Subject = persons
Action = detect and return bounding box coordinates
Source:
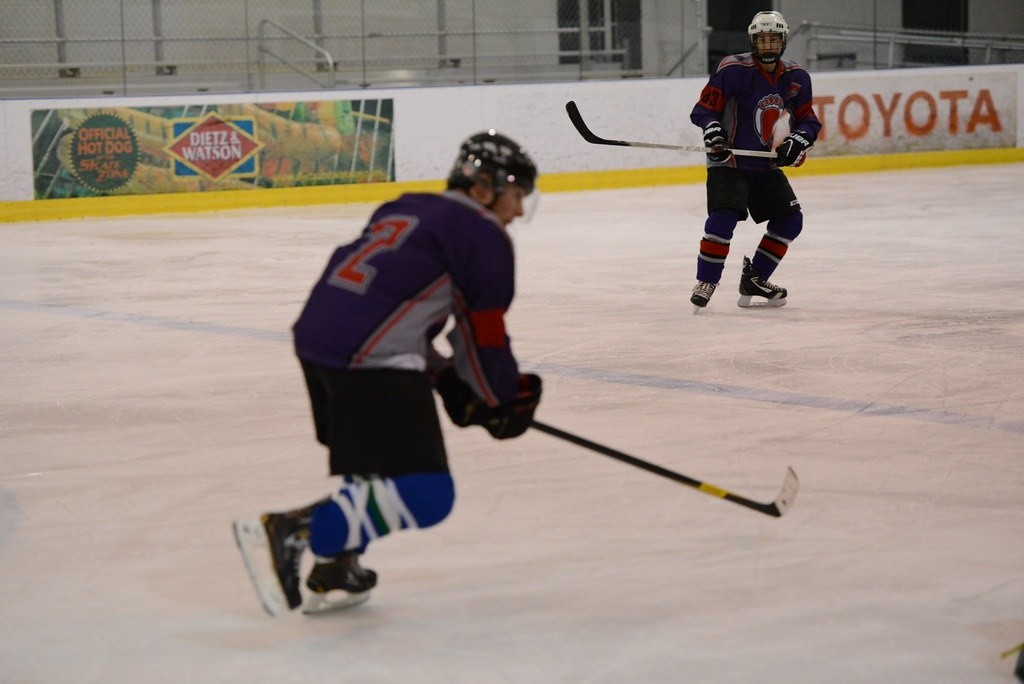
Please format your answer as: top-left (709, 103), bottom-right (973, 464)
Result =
top-left (233, 132), bottom-right (543, 619)
top-left (689, 11), bottom-right (823, 317)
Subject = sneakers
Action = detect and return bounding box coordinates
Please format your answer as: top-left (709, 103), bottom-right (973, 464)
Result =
top-left (689, 280), bottom-right (716, 308)
top-left (300, 553), bottom-right (379, 614)
top-left (232, 512), bottom-right (310, 615)
top-left (737, 255), bottom-right (788, 308)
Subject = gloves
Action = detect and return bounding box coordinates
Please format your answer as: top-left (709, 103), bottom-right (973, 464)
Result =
top-left (702, 120), bottom-right (733, 164)
top-left (431, 356), bottom-right (484, 428)
top-left (484, 372), bottom-right (543, 439)
top-left (775, 129), bottom-right (814, 168)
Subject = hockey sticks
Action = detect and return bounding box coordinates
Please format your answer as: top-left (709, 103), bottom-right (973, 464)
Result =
top-left (527, 417), bottom-right (799, 519)
top-left (565, 98), bottom-right (778, 159)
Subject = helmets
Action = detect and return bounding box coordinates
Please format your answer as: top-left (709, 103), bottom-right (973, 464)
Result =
top-left (447, 131), bottom-right (538, 196)
top-left (748, 10), bottom-right (791, 65)
